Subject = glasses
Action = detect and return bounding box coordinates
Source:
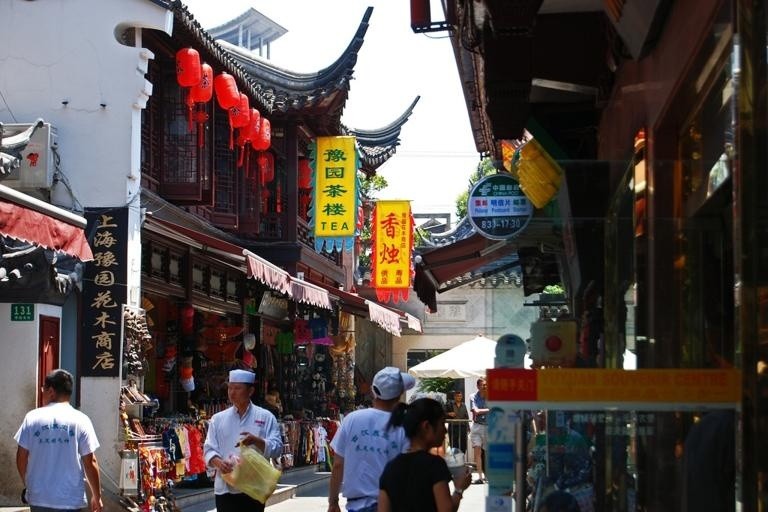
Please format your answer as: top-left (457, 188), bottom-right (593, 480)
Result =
top-left (40, 385), bottom-right (45, 393)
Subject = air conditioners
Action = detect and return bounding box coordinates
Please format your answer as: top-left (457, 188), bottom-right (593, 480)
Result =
top-left (0, 122), bottom-right (59, 191)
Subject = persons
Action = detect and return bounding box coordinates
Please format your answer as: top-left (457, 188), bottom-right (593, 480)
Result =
top-left (442, 390), bottom-right (471, 457)
top-left (467, 376), bottom-right (490, 484)
top-left (372, 395), bottom-right (474, 512)
top-left (532, 489), bottom-right (581, 511)
top-left (325, 366), bottom-right (418, 512)
top-left (8, 368), bottom-right (104, 511)
top-left (200, 368), bottom-right (285, 512)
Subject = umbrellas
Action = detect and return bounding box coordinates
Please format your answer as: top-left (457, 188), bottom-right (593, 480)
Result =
top-left (406, 332), bottom-right (535, 384)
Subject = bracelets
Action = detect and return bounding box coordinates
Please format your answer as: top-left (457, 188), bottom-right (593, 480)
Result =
top-left (328, 497), bottom-right (339, 505)
top-left (454, 490), bottom-right (463, 499)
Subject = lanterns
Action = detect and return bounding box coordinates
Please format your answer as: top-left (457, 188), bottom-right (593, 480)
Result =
top-left (174, 46), bottom-right (201, 132)
top-left (214, 70), bottom-right (240, 152)
top-left (255, 151), bottom-right (275, 217)
top-left (353, 205), bottom-right (365, 255)
top-left (297, 157), bottom-right (313, 221)
top-left (228, 91), bottom-right (250, 168)
top-left (188, 61), bottom-right (214, 149)
top-left (249, 117), bottom-right (271, 189)
top-left (237, 107), bottom-right (262, 178)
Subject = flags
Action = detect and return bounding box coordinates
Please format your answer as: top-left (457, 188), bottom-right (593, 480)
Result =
top-left (307, 135), bottom-right (363, 256)
top-left (370, 199), bottom-right (416, 304)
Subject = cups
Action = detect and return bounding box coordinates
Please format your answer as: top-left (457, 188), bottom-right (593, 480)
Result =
top-left (442, 446), bottom-right (467, 479)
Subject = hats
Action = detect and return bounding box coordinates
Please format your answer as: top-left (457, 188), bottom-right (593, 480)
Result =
top-left (242, 333), bottom-right (255, 352)
top-left (160, 307), bottom-right (195, 392)
top-left (228, 369), bottom-right (255, 384)
top-left (371, 366), bottom-right (416, 400)
